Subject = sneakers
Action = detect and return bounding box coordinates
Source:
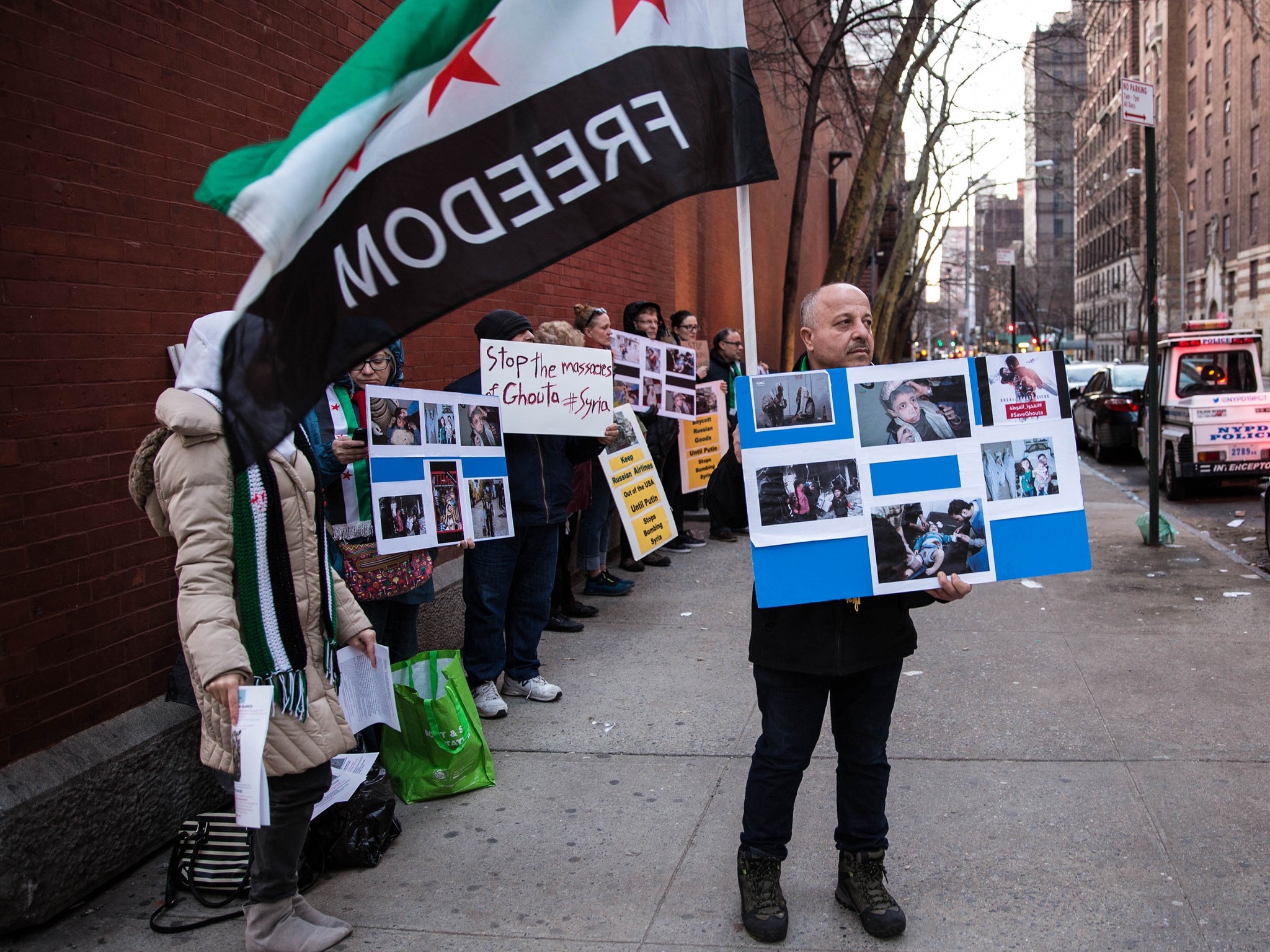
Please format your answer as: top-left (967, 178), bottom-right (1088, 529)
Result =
top-left (470, 681), bottom-right (508, 720)
top-left (835, 848), bottom-right (907, 939)
top-left (737, 842), bottom-right (789, 942)
top-left (500, 670), bottom-right (563, 703)
top-left (583, 572), bottom-right (632, 596)
top-left (678, 530), bottom-right (707, 547)
top-left (658, 538), bottom-right (692, 553)
top-left (601, 569), bottom-right (636, 588)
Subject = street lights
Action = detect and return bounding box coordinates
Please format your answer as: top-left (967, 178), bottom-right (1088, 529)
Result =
top-left (1126, 168), bottom-right (1187, 326)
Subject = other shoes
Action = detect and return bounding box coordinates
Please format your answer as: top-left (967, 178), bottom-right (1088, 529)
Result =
top-left (562, 600), bottom-right (600, 617)
top-left (620, 556), bottom-right (645, 572)
top-left (709, 531), bottom-right (738, 542)
top-left (543, 613), bottom-right (585, 631)
top-left (642, 551), bottom-right (672, 567)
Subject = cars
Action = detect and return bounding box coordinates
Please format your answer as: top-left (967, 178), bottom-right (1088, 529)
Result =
top-left (1064, 359), bottom-right (1227, 463)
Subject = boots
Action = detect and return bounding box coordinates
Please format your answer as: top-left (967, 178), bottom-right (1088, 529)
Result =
top-left (291, 888), bottom-right (354, 935)
top-left (241, 898), bottom-right (350, 952)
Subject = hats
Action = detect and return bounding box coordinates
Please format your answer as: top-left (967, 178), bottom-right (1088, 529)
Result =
top-left (474, 310), bottom-right (534, 344)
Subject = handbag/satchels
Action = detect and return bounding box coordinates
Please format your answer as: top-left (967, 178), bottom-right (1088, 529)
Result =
top-left (381, 649), bottom-right (495, 805)
top-left (149, 811), bottom-right (254, 935)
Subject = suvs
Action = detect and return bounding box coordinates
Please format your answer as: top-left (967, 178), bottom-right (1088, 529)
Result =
top-left (1139, 318), bottom-right (1270, 503)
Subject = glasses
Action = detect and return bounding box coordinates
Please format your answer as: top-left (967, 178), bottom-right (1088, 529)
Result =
top-left (585, 308), bottom-right (608, 325)
top-left (352, 355), bottom-right (391, 370)
top-left (634, 320), bottom-right (659, 324)
top-left (723, 341), bottom-right (743, 346)
top-left (679, 325), bottom-right (702, 331)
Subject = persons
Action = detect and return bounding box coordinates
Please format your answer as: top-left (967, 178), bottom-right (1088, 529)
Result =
top-left (668, 347), bottom-right (694, 375)
top-left (696, 388), bottom-right (718, 414)
top-left (287, 339), bottom-right (475, 663)
top-left (1019, 454), bottom-right (1052, 498)
top-left (370, 397), bottom-right (421, 445)
top-left (479, 476), bottom-right (505, 538)
top-left (759, 467), bottom-right (855, 528)
top-left (657, 310), bottom-right (707, 552)
top-left (437, 408), bottom-right (462, 531)
top-left (467, 404), bottom-right (500, 447)
top-left (871, 498), bottom-right (990, 584)
top-left (381, 501), bottom-right (423, 540)
top-left (643, 347), bottom-right (660, 406)
top-left (616, 339), bottom-right (637, 406)
top-left (616, 300), bottom-right (672, 571)
top-left (528, 319), bottom-right (598, 633)
top-left (881, 376), bottom-right (970, 444)
top-left (762, 384), bottom-right (814, 432)
top-left (706, 282), bottom-right (972, 946)
top-left (126, 312), bottom-right (376, 952)
top-left (439, 309), bottom-right (564, 721)
top-left (998, 356), bottom-right (1043, 424)
top-left (670, 391), bottom-right (695, 416)
top-left (570, 303), bottom-right (636, 598)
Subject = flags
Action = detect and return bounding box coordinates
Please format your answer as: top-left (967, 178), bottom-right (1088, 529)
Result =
top-left (193, 0), bottom-right (782, 476)
